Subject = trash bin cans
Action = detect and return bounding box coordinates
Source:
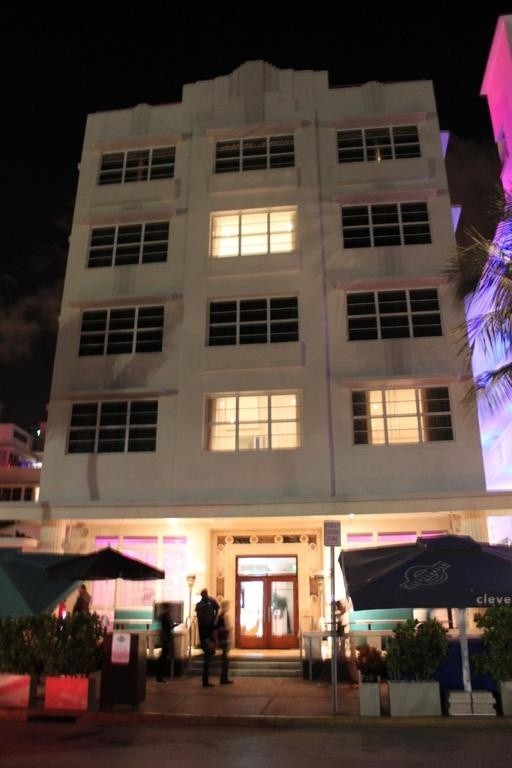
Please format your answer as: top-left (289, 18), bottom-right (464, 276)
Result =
top-left (99, 633), bottom-right (147, 713)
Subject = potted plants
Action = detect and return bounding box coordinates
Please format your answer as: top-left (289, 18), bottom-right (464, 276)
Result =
top-left (0, 607), bottom-right (107, 714)
top-left (352, 600), bottom-right (512, 720)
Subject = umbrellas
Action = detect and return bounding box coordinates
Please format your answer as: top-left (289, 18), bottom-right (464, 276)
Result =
top-left (1, 548), bottom-right (166, 620)
top-left (337, 534), bottom-right (512, 689)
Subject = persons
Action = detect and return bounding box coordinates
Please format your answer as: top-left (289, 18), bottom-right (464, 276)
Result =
top-left (426, 608), bottom-right (433, 620)
top-left (195, 588), bottom-right (234, 688)
top-left (73, 585), bottom-right (91, 616)
top-left (334, 600), bottom-right (347, 634)
top-left (271, 591), bottom-right (288, 618)
top-left (153, 601), bottom-right (180, 683)
